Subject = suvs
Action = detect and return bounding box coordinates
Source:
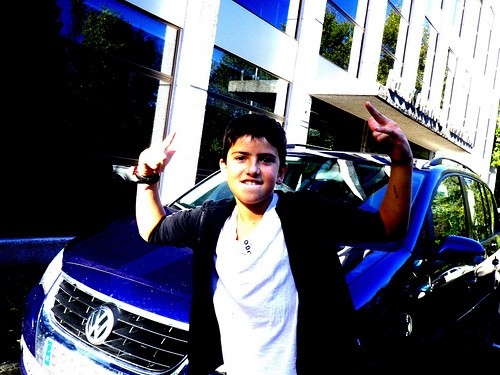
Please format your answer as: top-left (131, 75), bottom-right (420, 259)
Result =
top-left (17, 139), bottom-right (500, 375)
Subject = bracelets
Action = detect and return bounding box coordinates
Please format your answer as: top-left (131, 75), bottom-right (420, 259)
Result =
top-left (132, 169), bottom-right (161, 185)
top-left (388, 156), bottom-right (417, 169)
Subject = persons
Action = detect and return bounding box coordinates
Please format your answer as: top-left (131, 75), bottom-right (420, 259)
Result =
top-left (126, 100), bottom-right (413, 374)
